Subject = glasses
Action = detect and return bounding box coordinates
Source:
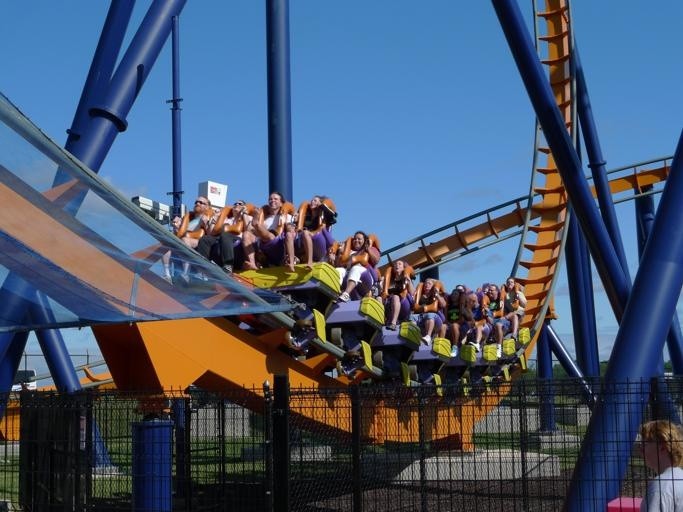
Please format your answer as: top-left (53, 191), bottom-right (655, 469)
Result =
top-left (195, 201), bottom-right (206, 205)
top-left (234, 203), bottom-right (243, 206)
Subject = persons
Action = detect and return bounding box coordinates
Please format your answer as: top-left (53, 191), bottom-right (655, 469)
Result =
top-left (636, 421), bottom-right (683, 512)
top-left (161, 191), bottom-right (529, 370)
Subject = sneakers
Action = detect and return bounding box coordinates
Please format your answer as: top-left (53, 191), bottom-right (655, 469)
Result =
top-left (512, 334), bottom-right (517, 341)
top-left (339, 293), bottom-right (349, 301)
top-left (497, 344), bottom-right (502, 358)
top-left (469, 342), bottom-right (480, 353)
top-left (223, 265), bottom-right (232, 272)
top-left (162, 272), bottom-right (208, 283)
top-left (422, 335), bottom-right (431, 345)
top-left (386, 323), bottom-right (395, 330)
top-left (451, 345), bottom-right (458, 357)
